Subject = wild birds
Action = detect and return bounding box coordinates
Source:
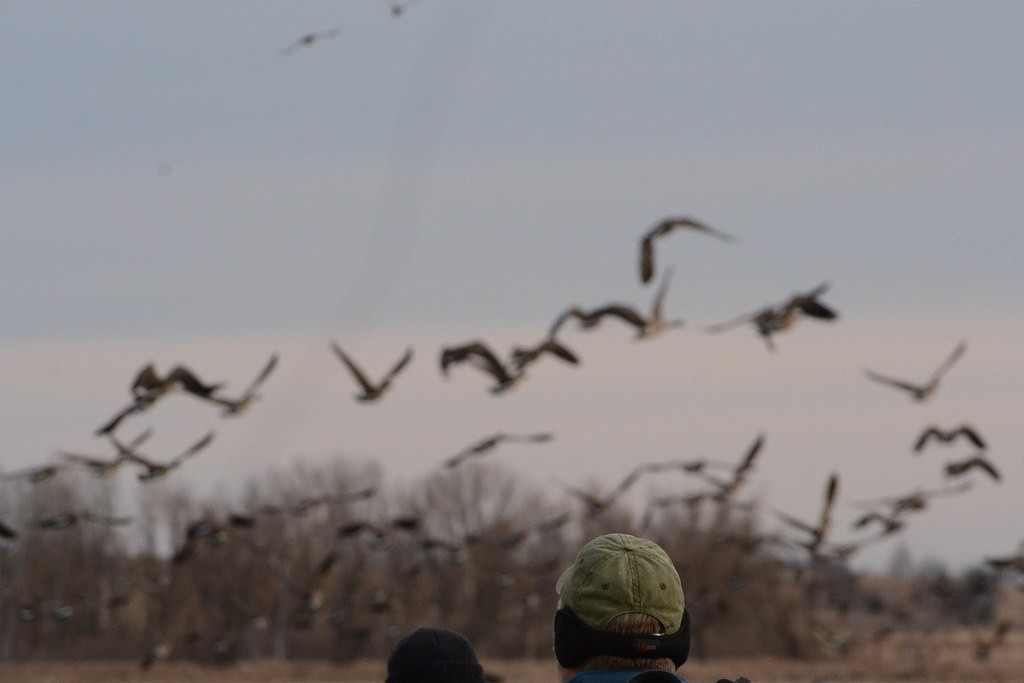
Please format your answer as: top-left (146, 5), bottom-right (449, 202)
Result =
top-left (278, 0), bottom-right (416, 59)
top-left (4, 213), bottom-right (1024, 669)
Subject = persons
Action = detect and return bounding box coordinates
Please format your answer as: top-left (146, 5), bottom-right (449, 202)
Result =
top-left (552, 532), bottom-right (692, 683)
top-left (382, 624), bottom-right (487, 683)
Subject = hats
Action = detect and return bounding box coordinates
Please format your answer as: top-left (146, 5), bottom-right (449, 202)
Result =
top-left (554, 532), bottom-right (685, 634)
top-left (385, 627), bottom-right (484, 683)
